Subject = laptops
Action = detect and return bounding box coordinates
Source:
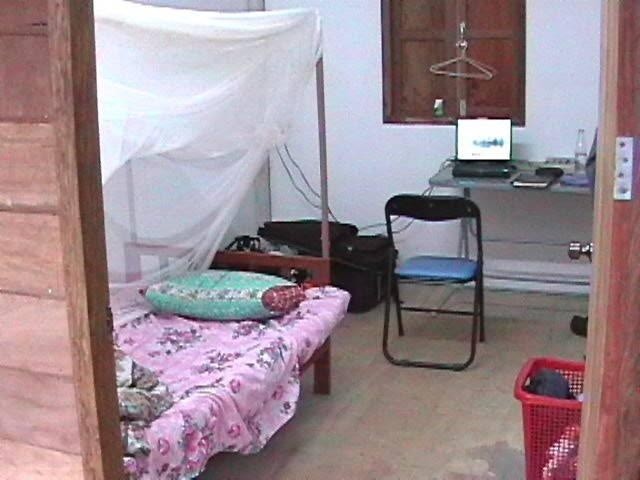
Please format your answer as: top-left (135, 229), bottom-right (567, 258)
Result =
top-left (452, 117), bottom-right (512, 177)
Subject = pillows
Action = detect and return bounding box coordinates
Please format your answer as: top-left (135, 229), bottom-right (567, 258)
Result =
top-left (138, 270), bottom-right (306, 321)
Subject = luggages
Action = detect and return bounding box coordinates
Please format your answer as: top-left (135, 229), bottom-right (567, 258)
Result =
top-left (254, 223), bottom-right (397, 314)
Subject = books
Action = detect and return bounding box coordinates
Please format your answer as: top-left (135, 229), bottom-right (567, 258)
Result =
top-left (510, 172), bottom-right (555, 189)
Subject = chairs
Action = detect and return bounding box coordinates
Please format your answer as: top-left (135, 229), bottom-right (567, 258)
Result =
top-left (382, 193), bottom-right (486, 371)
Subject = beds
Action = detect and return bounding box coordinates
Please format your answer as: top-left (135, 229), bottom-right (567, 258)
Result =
top-left (108, 243), bottom-right (332, 480)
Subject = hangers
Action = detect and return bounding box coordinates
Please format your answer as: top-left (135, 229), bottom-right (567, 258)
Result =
top-left (429, 40), bottom-right (498, 80)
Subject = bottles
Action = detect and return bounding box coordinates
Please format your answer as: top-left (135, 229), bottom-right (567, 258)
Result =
top-left (574, 129), bottom-right (587, 175)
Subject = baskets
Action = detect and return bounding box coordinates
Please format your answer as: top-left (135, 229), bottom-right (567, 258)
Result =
top-left (515, 357), bottom-right (585, 480)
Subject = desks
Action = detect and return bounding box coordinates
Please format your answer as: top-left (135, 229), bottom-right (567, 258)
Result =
top-left (428, 159), bottom-right (593, 318)
